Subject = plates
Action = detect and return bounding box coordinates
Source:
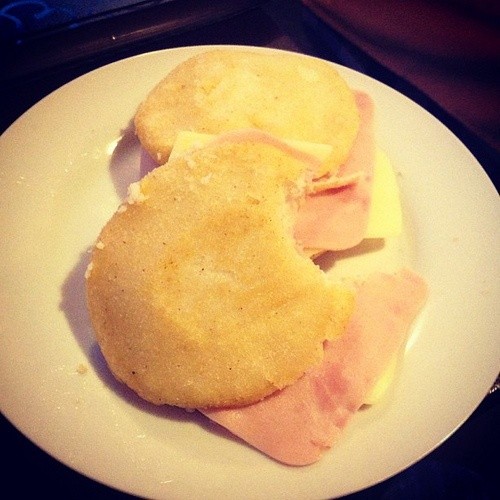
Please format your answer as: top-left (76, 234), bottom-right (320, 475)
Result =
top-left (0, 44), bottom-right (500, 500)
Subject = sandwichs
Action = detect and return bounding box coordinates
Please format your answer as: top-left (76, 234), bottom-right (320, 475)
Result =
top-left (86, 129), bottom-right (430, 465)
top-left (132, 48), bottom-right (403, 256)
